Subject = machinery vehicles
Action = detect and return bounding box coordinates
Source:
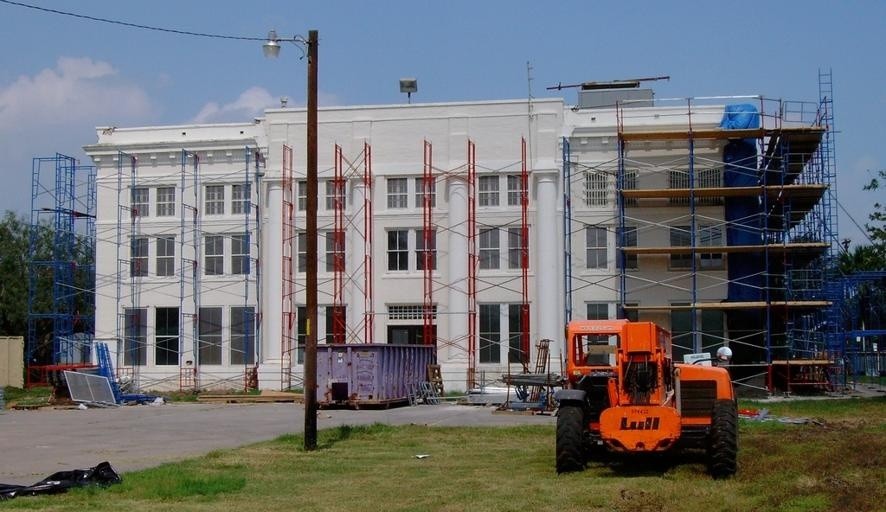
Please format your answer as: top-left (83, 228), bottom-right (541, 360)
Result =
top-left (555, 316), bottom-right (738, 478)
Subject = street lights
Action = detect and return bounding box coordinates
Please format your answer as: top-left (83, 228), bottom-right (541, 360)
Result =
top-left (263, 28), bottom-right (318, 452)
